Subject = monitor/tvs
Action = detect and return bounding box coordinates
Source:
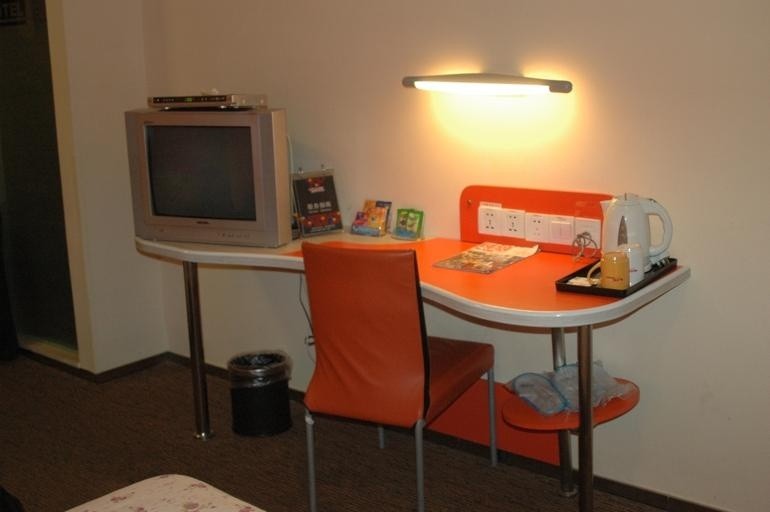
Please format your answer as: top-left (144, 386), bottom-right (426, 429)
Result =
top-left (122, 107), bottom-right (295, 249)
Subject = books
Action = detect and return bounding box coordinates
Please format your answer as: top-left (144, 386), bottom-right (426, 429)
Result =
top-left (430, 241), bottom-right (541, 275)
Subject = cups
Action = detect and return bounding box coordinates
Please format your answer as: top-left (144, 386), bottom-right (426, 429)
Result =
top-left (588, 251), bottom-right (630, 292)
top-left (616, 243), bottom-right (644, 288)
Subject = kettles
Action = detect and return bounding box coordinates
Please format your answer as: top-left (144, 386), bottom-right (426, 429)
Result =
top-left (599, 191), bottom-right (673, 272)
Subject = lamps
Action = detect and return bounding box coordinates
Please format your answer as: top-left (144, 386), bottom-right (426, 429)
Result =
top-left (398, 71), bottom-right (574, 95)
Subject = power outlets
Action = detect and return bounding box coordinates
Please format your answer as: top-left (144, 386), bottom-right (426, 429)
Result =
top-left (477, 205), bottom-right (547, 244)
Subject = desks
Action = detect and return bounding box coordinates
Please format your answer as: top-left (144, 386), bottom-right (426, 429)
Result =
top-left (132, 226), bottom-right (691, 512)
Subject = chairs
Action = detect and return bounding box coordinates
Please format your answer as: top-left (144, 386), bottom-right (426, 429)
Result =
top-left (298, 240), bottom-right (501, 508)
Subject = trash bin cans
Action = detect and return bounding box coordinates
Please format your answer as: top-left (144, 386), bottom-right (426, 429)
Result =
top-left (228, 349), bottom-right (292, 436)
